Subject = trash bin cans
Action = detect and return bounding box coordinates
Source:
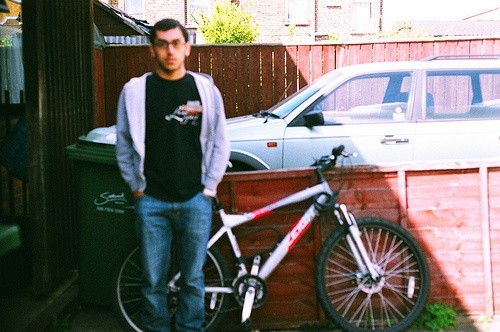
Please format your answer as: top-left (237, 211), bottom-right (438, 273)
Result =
top-left (65, 127), bottom-right (176, 313)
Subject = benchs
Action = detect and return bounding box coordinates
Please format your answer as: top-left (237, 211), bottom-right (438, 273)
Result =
top-left (470, 101), bottom-right (500, 118)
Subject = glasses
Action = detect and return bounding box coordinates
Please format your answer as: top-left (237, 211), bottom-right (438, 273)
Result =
top-left (153, 39), bottom-right (186, 48)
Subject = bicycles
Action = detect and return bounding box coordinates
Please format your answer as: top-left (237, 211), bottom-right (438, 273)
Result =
top-left (115, 144), bottom-right (430, 332)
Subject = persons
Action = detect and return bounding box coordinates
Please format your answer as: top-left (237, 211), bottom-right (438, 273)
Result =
top-left (116, 19), bottom-right (231, 332)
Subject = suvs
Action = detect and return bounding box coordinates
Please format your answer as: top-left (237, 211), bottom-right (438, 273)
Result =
top-left (65, 53), bottom-right (500, 186)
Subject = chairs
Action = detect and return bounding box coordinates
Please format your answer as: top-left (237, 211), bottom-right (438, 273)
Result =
top-left (396, 91), bottom-right (434, 120)
